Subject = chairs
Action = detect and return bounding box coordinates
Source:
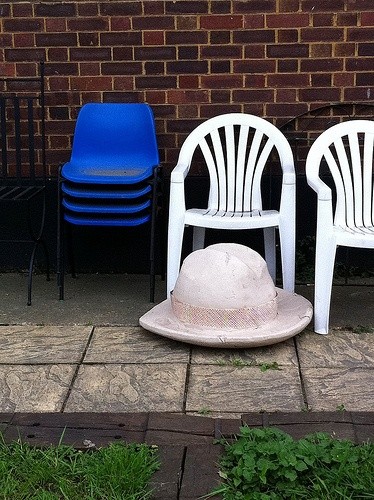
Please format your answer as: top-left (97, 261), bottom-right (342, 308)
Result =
top-left (166, 113), bottom-right (296, 300)
top-left (306, 119), bottom-right (374, 335)
top-left (0, 53), bottom-right (166, 305)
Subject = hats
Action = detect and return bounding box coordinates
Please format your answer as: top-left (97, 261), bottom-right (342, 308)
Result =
top-left (139, 243), bottom-right (313, 348)
